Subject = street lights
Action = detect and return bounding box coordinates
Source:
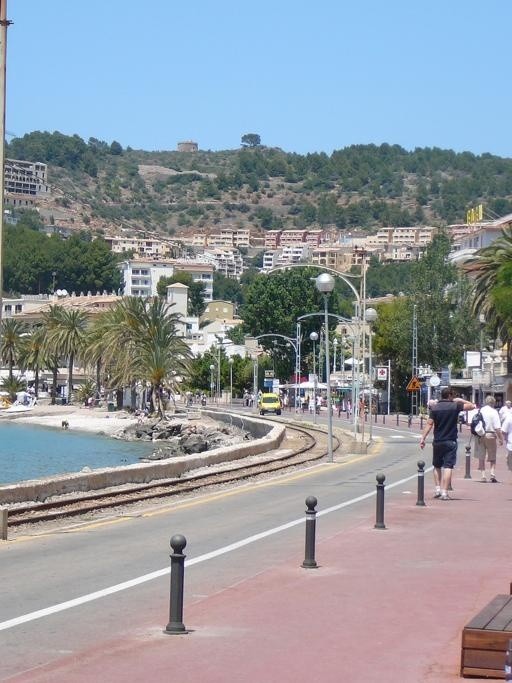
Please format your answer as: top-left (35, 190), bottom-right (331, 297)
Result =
top-left (364, 306), bottom-right (379, 439)
top-left (309, 331), bottom-right (319, 424)
top-left (227, 357), bottom-right (233, 409)
top-left (315, 271), bottom-right (338, 463)
top-left (209, 364), bottom-right (215, 406)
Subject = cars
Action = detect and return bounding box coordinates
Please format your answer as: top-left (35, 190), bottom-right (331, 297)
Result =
top-left (375, 402), bottom-right (394, 414)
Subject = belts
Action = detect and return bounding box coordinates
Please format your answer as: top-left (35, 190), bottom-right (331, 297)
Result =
top-left (485, 431), bottom-right (496, 433)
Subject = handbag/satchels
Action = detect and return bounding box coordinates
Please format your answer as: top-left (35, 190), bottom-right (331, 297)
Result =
top-left (470, 412), bottom-right (486, 436)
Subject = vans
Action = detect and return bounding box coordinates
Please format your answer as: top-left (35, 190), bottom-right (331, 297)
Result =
top-left (259, 392), bottom-right (282, 415)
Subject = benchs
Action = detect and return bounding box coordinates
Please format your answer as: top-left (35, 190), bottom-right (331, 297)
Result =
top-left (459, 592), bottom-right (512, 680)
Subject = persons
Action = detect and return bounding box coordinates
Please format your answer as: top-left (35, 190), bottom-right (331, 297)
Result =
top-left (61, 420), bottom-right (70, 430)
top-left (278, 390), bottom-right (288, 412)
top-left (84, 392), bottom-right (95, 408)
top-left (426, 395), bottom-right (512, 490)
top-left (244, 389), bottom-right (262, 407)
top-left (295, 394), bottom-right (307, 413)
top-left (184, 389), bottom-right (207, 407)
top-left (29, 394), bottom-right (37, 408)
top-left (346, 397), bottom-right (366, 419)
top-left (420, 388), bottom-right (474, 500)
top-left (309, 393), bottom-right (323, 415)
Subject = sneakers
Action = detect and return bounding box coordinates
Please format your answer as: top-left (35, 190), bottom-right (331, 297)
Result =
top-left (482, 475), bottom-right (497, 483)
top-left (434, 492), bottom-right (451, 501)
